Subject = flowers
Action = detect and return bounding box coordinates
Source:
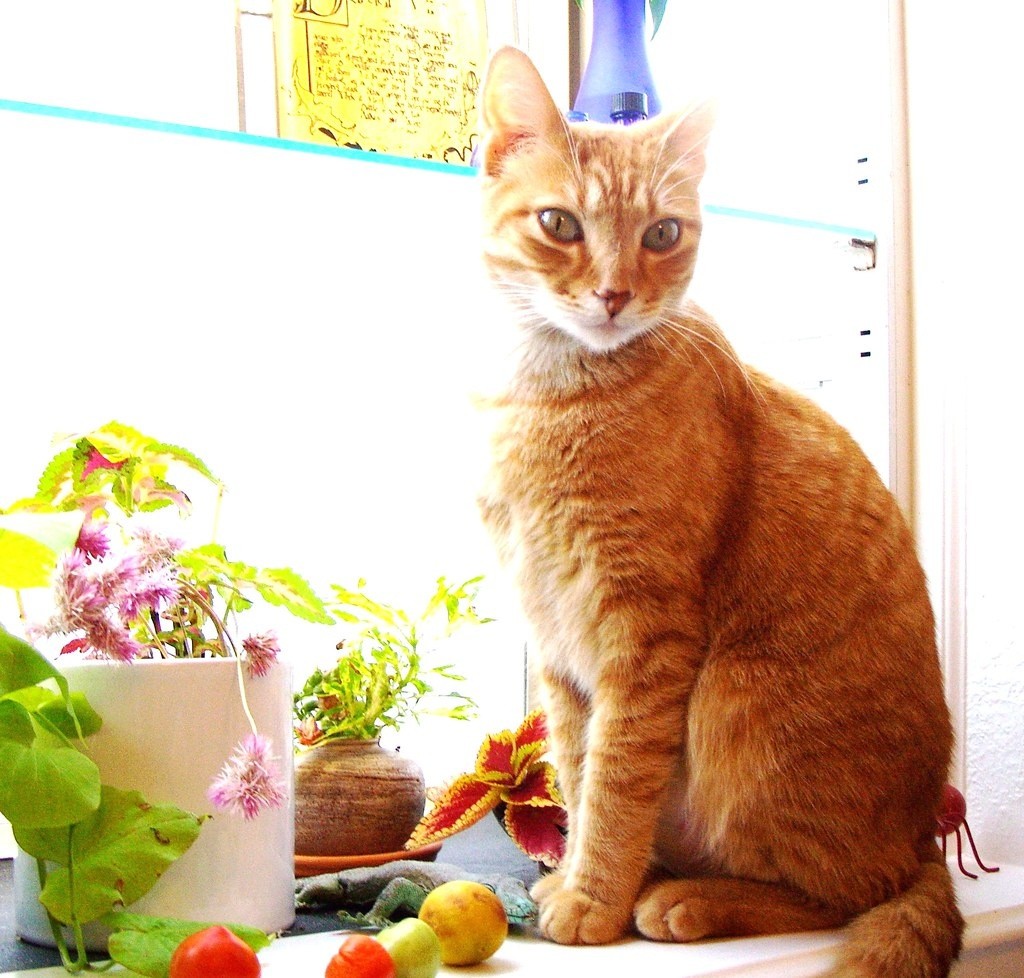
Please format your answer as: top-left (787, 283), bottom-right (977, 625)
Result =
top-left (286, 576), bottom-right (498, 747)
top-left (45, 442), bottom-right (284, 824)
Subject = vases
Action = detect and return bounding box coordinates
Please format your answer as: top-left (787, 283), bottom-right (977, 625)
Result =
top-left (292, 740), bottom-right (443, 876)
top-left (14, 660), bottom-right (296, 946)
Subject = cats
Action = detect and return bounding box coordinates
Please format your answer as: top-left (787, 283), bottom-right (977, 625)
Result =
top-left (474, 45), bottom-right (969, 978)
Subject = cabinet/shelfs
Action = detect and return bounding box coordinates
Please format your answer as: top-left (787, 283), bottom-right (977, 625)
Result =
top-left (0, 861), bottom-right (1024, 978)
